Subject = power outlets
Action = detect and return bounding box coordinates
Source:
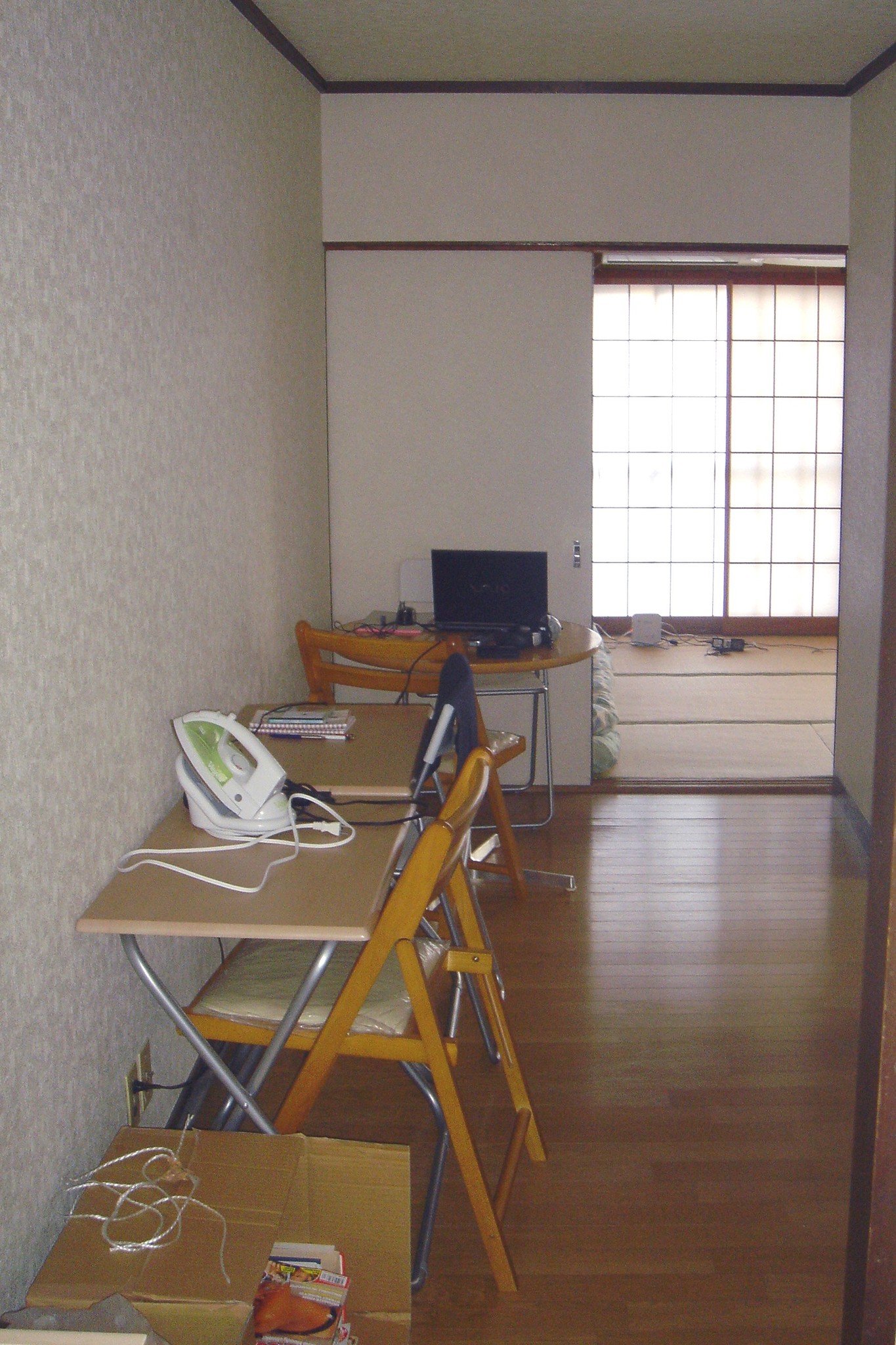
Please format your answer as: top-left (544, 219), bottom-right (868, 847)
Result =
top-left (125, 1063), bottom-right (141, 1127)
top-left (137, 1037), bottom-right (154, 1113)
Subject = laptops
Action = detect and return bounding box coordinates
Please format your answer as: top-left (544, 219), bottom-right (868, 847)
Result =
top-left (431, 549), bottom-right (548, 632)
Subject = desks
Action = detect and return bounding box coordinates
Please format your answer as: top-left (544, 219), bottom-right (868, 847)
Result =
top-left (229, 702), bottom-right (507, 1062)
top-left (332, 620), bottom-right (603, 826)
top-left (73, 794), bottom-right (449, 1293)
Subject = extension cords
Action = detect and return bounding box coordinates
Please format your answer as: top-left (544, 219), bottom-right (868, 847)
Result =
top-left (710, 638), bottom-right (745, 651)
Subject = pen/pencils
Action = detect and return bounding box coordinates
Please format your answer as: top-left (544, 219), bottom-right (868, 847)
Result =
top-left (290, 733), bottom-right (355, 740)
top-left (270, 734), bottom-right (324, 739)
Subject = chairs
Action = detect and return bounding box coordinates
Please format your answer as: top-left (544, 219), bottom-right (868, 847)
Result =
top-left (176, 734), bottom-right (545, 1293)
top-left (295, 620), bottom-right (526, 897)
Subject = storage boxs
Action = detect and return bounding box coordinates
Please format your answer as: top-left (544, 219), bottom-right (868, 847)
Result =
top-left (24, 1126), bottom-right (412, 1345)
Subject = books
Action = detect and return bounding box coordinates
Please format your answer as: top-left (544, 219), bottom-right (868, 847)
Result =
top-left (357, 610), bottom-right (434, 634)
top-left (249, 704), bottom-right (356, 735)
